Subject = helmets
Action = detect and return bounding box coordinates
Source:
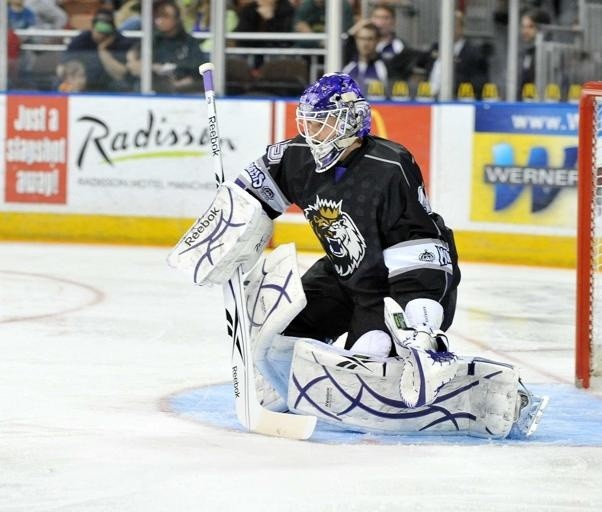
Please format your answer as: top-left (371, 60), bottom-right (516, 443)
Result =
top-left (295, 72), bottom-right (370, 172)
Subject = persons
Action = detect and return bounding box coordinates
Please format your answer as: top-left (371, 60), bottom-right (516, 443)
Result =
top-left (171, 71), bottom-right (551, 436)
top-left (1, 1), bottom-right (563, 100)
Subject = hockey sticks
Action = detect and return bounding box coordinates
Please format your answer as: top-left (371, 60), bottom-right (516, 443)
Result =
top-left (197, 63), bottom-right (318, 440)
